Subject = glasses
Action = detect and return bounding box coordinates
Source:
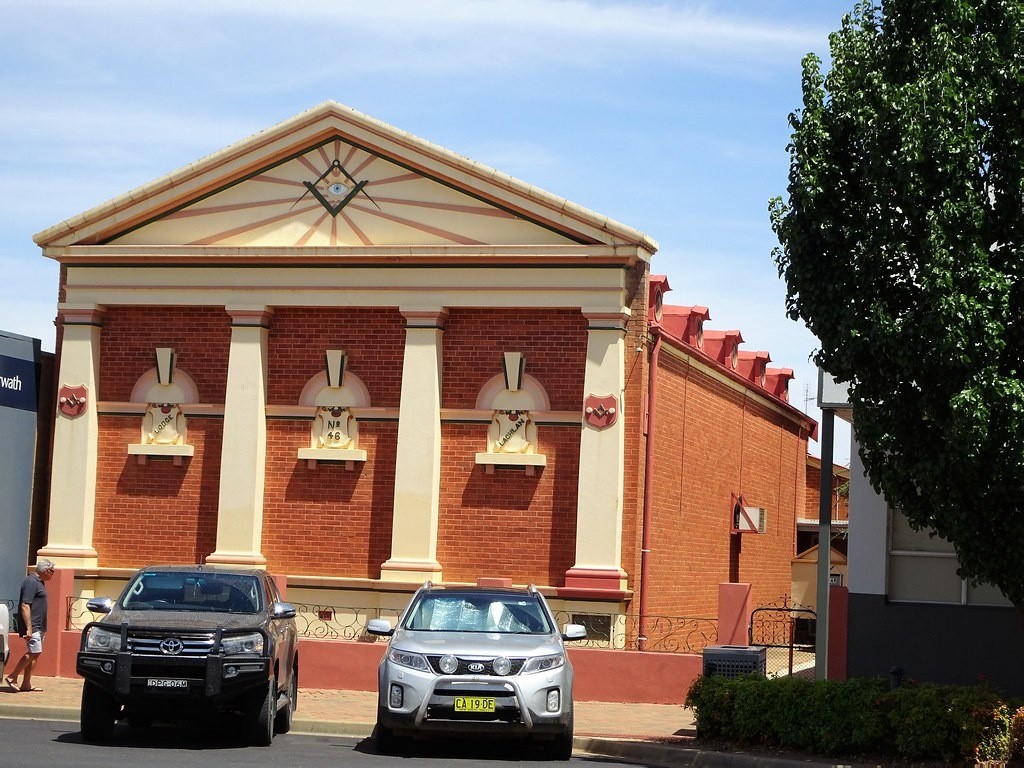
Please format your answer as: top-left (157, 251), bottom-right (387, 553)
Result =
top-left (48, 568), bottom-right (55, 574)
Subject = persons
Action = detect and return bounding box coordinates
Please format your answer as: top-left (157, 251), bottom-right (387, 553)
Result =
top-left (6, 559), bottom-right (55, 691)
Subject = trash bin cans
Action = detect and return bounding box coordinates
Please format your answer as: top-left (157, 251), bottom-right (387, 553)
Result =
top-left (703, 645), bottom-right (767, 677)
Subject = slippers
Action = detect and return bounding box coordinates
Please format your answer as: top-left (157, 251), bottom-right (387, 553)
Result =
top-left (19, 686), bottom-right (43, 692)
top-left (5, 677), bottom-right (20, 692)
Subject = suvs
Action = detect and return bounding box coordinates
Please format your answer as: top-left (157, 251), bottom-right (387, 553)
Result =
top-left (0, 603), bottom-right (9, 682)
top-left (367, 580), bottom-right (588, 759)
top-left (76, 563), bottom-right (299, 746)
